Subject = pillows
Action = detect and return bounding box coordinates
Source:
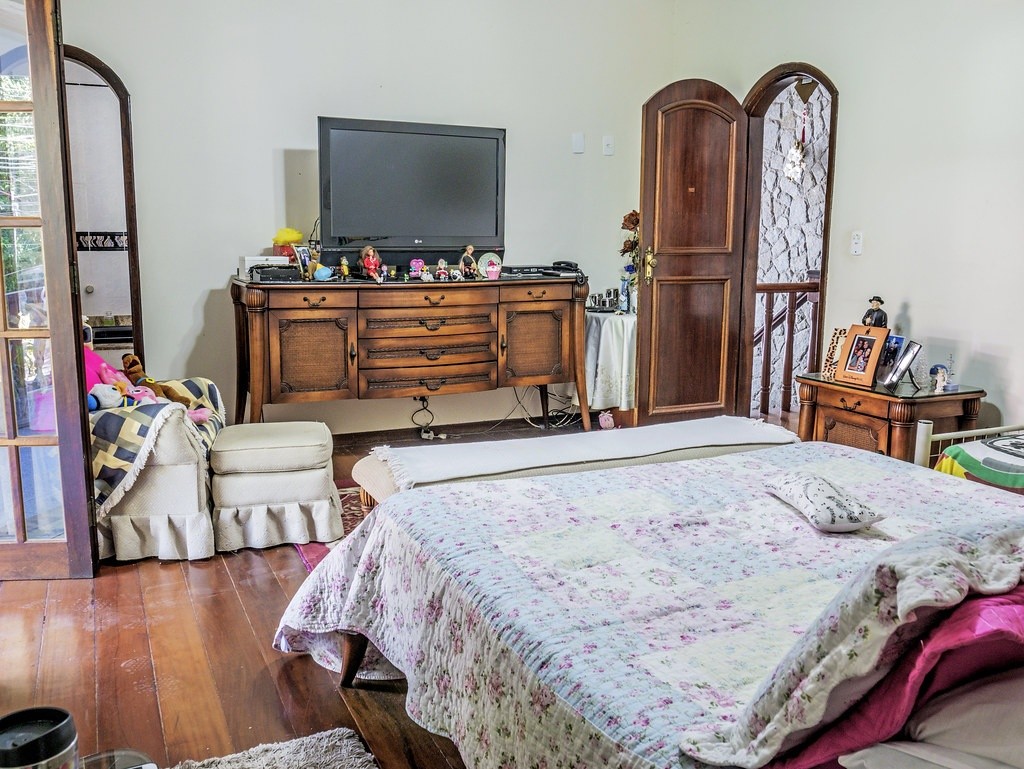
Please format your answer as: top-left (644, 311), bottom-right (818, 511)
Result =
top-left (904, 666), bottom-right (1023, 769)
top-left (759, 583), bottom-right (1023, 768)
top-left (762, 469), bottom-right (889, 533)
top-left (833, 741), bottom-right (1023, 769)
top-left (675, 515), bottom-right (1023, 768)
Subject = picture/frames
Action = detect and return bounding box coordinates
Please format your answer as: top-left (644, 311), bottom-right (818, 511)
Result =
top-left (884, 341), bottom-right (922, 394)
top-left (823, 326), bottom-right (851, 377)
top-left (876, 334), bottom-right (907, 383)
top-left (834, 323), bottom-right (892, 387)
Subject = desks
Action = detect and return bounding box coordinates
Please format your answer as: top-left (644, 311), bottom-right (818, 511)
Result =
top-left (584, 308), bottom-right (637, 428)
top-left (793, 371), bottom-right (988, 472)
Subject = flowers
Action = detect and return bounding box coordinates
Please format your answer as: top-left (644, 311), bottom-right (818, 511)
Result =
top-left (619, 209), bottom-right (640, 290)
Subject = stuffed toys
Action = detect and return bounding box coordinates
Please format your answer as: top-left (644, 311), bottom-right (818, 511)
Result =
top-left (81, 314), bottom-right (213, 427)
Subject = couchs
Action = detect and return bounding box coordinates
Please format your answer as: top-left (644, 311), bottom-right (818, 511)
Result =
top-left (8, 299), bottom-right (227, 562)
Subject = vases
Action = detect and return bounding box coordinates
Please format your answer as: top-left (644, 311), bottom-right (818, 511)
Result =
top-left (627, 273), bottom-right (638, 313)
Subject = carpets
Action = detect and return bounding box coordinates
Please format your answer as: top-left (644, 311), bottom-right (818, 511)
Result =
top-left (291, 478), bottom-right (366, 574)
top-left (160, 726), bottom-right (379, 768)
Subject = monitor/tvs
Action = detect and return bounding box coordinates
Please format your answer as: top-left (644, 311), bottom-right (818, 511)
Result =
top-left (317, 116), bottom-right (507, 266)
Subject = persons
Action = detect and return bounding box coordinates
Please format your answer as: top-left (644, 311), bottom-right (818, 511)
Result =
top-left (379, 262), bottom-right (390, 283)
top-left (458, 244), bottom-right (479, 279)
top-left (862, 295), bottom-right (888, 329)
top-left (340, 255), bottom-right (350, 282)
top-left (360, 245), bottom-right (384, 284)
top-left (848, 337), bottom-right (872, 373)
top-left (296, 248), bottom-right (312, 273)
top-left (876, 339), bottom-right (900, 381)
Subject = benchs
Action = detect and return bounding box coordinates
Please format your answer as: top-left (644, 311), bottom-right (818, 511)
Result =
top-left (351, 414), bottom-right (804, 519)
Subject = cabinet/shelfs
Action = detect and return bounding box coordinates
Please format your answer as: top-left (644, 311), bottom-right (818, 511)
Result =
top-left (228, 273), bottom-right (593, 433)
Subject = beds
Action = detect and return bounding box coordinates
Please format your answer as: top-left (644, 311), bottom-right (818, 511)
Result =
top-left (273, 436), bottom-right (1024, 769)
top-left (914, 419), bottom-right (1024, 495)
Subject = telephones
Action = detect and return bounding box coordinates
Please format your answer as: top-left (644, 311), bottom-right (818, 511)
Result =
top-left (541, 260), bottom-right (589, 285)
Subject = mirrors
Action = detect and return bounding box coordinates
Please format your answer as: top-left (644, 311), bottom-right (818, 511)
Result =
top-left (0, 41), bottom-right (146, 376)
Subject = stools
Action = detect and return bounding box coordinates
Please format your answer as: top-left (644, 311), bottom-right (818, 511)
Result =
top-left (209, 419), bottom-right (345, 553)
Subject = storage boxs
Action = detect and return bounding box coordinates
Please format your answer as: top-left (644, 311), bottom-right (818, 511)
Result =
top-left (239, 255), bottom-right (288, 281)
